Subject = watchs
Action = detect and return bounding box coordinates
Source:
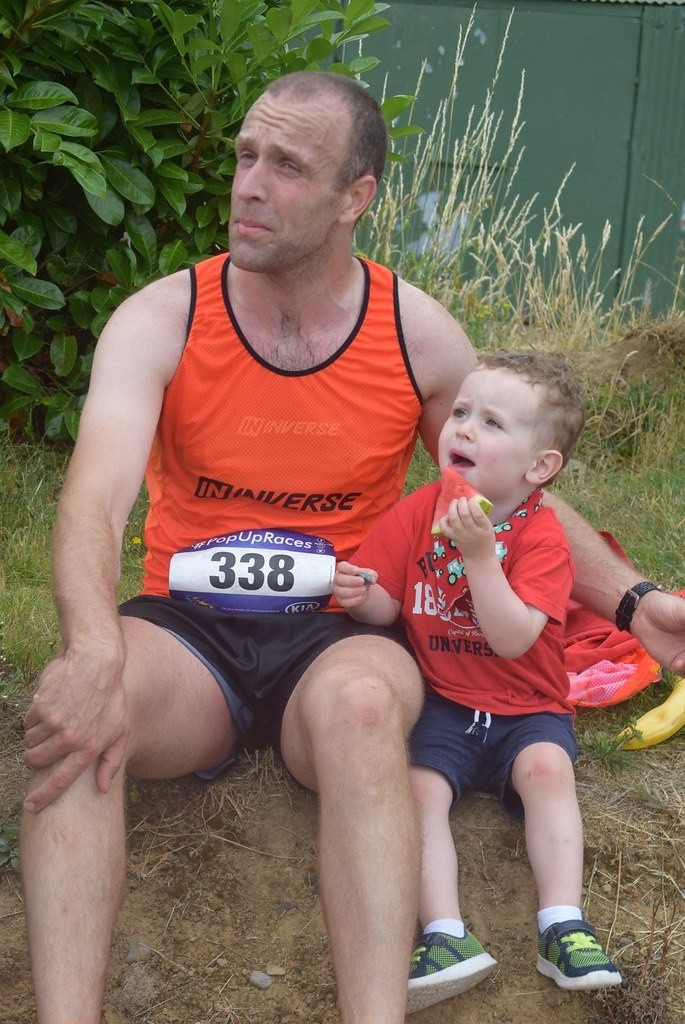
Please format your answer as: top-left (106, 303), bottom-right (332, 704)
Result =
top-left (612, 581), bottom-right (659, 635)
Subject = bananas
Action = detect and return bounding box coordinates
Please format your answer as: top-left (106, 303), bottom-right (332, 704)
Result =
top-left (614, 674), bottom-right (685, 751)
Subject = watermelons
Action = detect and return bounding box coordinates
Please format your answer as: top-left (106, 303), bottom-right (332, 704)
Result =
top-left (431, 467), bottom-right (494, 537)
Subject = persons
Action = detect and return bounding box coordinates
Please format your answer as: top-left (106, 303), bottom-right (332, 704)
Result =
top-left (16, 62), bottom-right (684, 1024)
top-left (328, 343), bottom-right (624, 1024)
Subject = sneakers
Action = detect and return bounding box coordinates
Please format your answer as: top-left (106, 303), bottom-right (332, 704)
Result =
top-left (405, 923), bottom-right (499, 1015)
top-left (536, 920), bottom-right (622, 990)
